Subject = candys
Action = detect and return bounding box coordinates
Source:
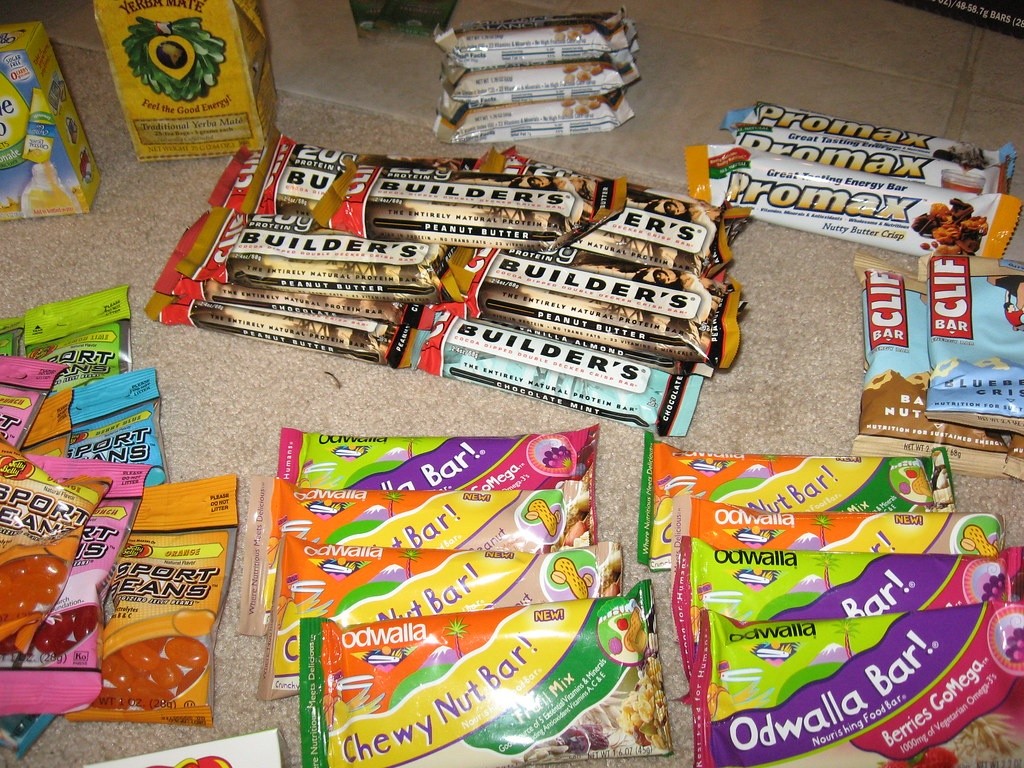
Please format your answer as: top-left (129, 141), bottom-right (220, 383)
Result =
top-left (0, 7), bottom-right (1024, 768)
